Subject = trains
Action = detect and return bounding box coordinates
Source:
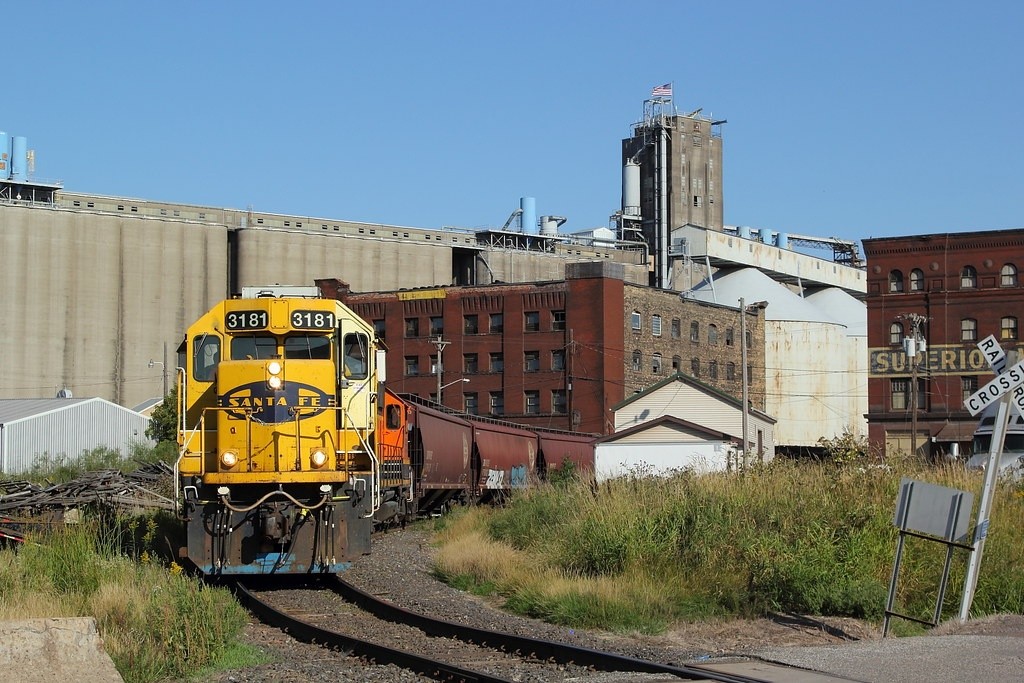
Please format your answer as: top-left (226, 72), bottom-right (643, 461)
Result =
top-left (176, 289), bottom-right (603, 581)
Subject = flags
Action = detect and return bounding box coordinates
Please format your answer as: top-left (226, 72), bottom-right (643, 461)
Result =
top-left (652, 83), bottom-right (672, 96)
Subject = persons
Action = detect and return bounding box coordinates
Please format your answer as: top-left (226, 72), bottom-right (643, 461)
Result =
top-left (205, 351), bottom-right (219, 378)
